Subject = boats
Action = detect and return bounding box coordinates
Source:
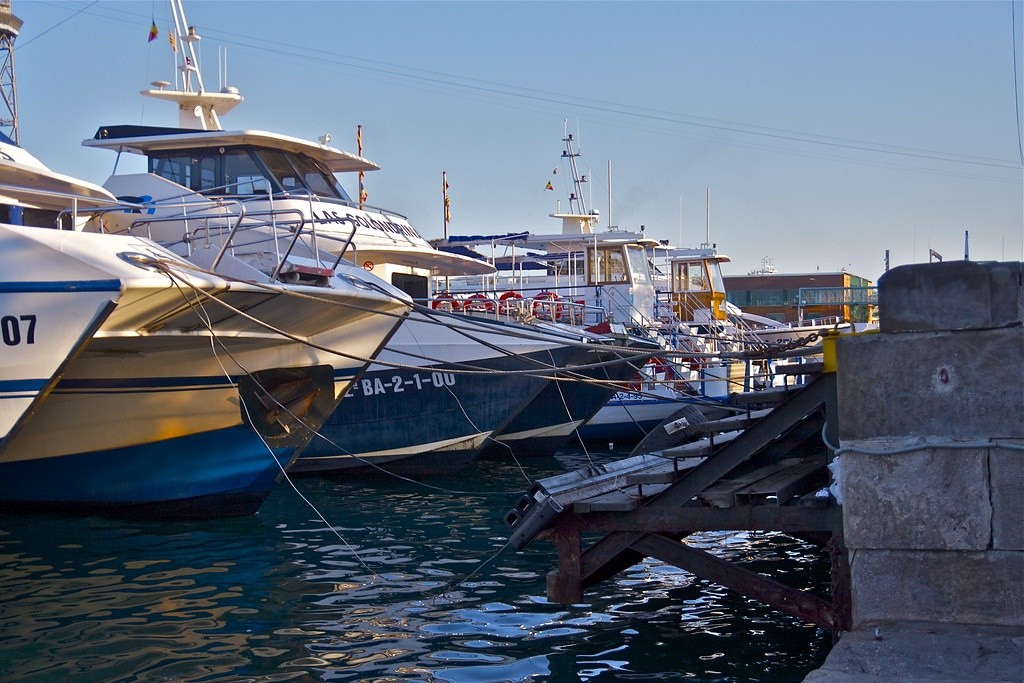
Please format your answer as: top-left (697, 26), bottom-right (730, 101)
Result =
top-left (1, 0), bottom-right (869, 525)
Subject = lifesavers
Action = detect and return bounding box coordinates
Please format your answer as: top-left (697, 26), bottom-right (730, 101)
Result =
top-left (497, 291), bottom-right (524, 314)
top-left (532, 291), bottom-right (563, 318)
top-left (568, 300), bottom-right (585, 316)
top-left (463, 294), bottom-right (493, 310)
top-left (432, 295), bottom-right (460, 311)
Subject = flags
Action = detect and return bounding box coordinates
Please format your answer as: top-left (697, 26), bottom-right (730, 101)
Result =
top-left (148, 19), bottom-right (159, 42)
top-left (168, 28), bottom-right (178, 52)
